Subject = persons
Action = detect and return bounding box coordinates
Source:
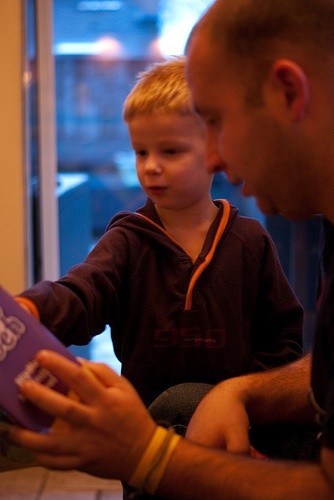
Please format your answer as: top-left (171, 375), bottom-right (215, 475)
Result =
top-left (0, 0), bottom-right (334, 500)
top-left (8, 57), bottom-right (304, 413)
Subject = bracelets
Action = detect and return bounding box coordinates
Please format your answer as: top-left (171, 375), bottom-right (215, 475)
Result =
top-left (126, 426), bottom-right (183, 494)
